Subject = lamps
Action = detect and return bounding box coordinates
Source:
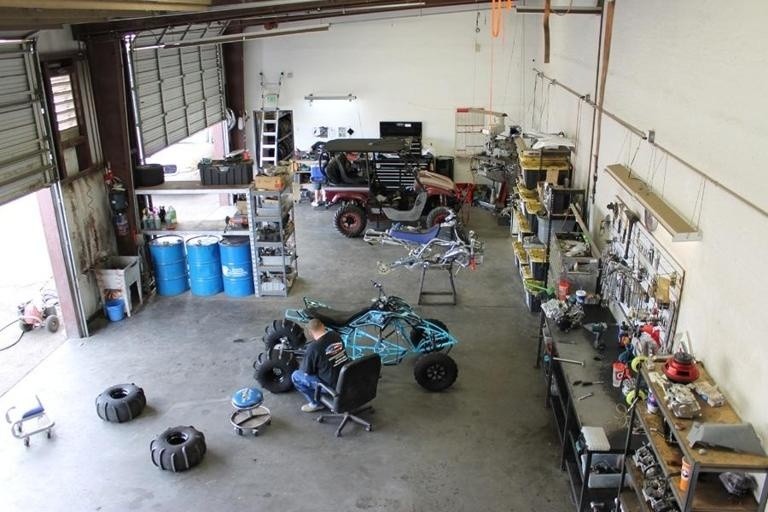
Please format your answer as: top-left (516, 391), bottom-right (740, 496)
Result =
top-left (605, 137), bottom-right (708, 244)
top-left (512, 0), bottom-right (602, 15)
top-left (158, 18), bottom-right (332, 51)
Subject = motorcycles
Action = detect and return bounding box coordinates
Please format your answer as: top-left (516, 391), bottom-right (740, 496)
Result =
top-left (251, 279), bottom-right (458, 398)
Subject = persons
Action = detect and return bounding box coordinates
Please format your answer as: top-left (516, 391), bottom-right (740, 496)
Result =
top-left (310, 184), bottom-right (327, 207)
top-left (290, 318), bottom-right (350, 413)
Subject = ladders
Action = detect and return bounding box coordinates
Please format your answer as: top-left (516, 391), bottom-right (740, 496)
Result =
top-left (259, 71), bottom-right (284, 168)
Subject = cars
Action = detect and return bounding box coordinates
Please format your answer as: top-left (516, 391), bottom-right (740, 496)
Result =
top-left (144, 129), bottom-right (215, 182)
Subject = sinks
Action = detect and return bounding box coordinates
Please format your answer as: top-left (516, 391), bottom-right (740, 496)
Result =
top-left (93, 255), bottom-right (141, 290)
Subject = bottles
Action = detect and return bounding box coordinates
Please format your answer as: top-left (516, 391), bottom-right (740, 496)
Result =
top-left (592, 319), bottom-right (603, 349)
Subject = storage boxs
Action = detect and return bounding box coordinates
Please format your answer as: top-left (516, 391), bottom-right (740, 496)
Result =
top-left (196, 157), bottom-right (255, 186)
top-left (505, 132), bottom-right (600, 315)
top-left (251, 169), bottom-right (298, 296)
top-left (579, 452), bottom-right (633, 488)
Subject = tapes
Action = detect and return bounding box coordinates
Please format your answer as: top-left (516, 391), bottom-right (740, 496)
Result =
top-left (631, 356), bottom-right (648, 373)
top-left (625, 389), bottom-right (646, 406)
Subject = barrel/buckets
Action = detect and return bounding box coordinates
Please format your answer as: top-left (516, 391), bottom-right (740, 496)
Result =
top-left (104, 299), bottom-right (125, 323)
top-left (146, 234), bottom-right (190, 298)
top-left (218, 236), bottom-right (255, 299)
top-left (185, 234), bottom-right (223, 298)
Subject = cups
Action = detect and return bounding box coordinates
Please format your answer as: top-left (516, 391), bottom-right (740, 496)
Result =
top-left (558, 282), bottom-right (570, 302)
top-left (680, 456), bottom-right (689, 491)
top-left (612, 362), bottom-right (624, 387)
top-left (575, 291), bottom-right (587, 304)
top-left (647, 387), bottom-right (658, 415)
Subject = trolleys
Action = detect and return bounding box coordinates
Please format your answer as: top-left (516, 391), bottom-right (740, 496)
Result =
top-left (1, 297), bottom-right (61, 353)
top-left (7, 395), bottom-right (54, 445)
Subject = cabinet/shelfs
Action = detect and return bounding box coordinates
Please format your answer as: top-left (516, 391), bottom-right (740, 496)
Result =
top-left (534, 301), bottom-right (656, 511)
top-left (134, 179), bottom-right (258, 297)
top-left (612, 359), bottom-right (768, 512)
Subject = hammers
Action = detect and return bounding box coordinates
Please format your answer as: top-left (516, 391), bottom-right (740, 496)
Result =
top-left (623, 210), bottom-right (639, 260)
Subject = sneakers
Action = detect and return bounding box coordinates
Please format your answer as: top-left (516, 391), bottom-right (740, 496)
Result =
top-left (300, 402), bottom-right (325, 412)
top-left (310, 202), bottom-right (319, 207)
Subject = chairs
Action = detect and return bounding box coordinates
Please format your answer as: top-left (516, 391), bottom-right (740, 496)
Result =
top-left (313, 353), bottom-right (382, 438)
top-left (335, 154), bottom-right (370, 186)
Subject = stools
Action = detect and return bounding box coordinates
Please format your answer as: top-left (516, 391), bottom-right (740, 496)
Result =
top-left (2, 395), bottom-right (55, 449)
top-left (417, 261), bottom-right (459, 307)
top-left (228, 386), bottom-right (271, 438)
